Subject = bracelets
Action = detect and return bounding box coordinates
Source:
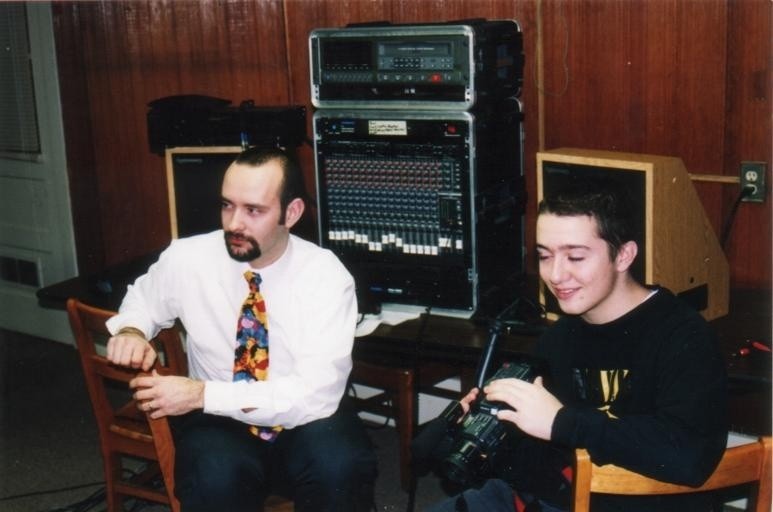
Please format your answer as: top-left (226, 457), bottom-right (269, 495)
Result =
top-left (116, 326), bottom-right (146, 339)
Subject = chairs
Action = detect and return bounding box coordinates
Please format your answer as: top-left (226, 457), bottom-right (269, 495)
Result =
top-left (571, 436), bottom-right (771, 511)
top-left (64, 295), bottom-right (295, 512)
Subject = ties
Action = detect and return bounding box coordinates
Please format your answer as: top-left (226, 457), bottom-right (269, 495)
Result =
top-left (231, 271), bottom-right (285, 441)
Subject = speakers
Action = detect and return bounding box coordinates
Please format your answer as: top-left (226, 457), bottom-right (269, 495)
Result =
top-left (535, 146), bottom-right (731, 324)
top-left (163, 144), bottom-right (287, 240)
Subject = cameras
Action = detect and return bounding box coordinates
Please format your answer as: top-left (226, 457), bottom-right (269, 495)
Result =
top-left (439, 361), bottom-right (533, 485)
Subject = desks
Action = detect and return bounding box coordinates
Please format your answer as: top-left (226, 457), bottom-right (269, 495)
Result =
top-left (33, 246), bottom-right (770, 487)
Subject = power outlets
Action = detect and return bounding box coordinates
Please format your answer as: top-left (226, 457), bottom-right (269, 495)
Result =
top-left (738, 161), bottom-right (767, 205)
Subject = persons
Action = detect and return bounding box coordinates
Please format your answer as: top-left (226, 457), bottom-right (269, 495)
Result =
top-left (431, 185), bottom-right (731, 510)
top-left (103, 144), bottom-right (375, 510)
